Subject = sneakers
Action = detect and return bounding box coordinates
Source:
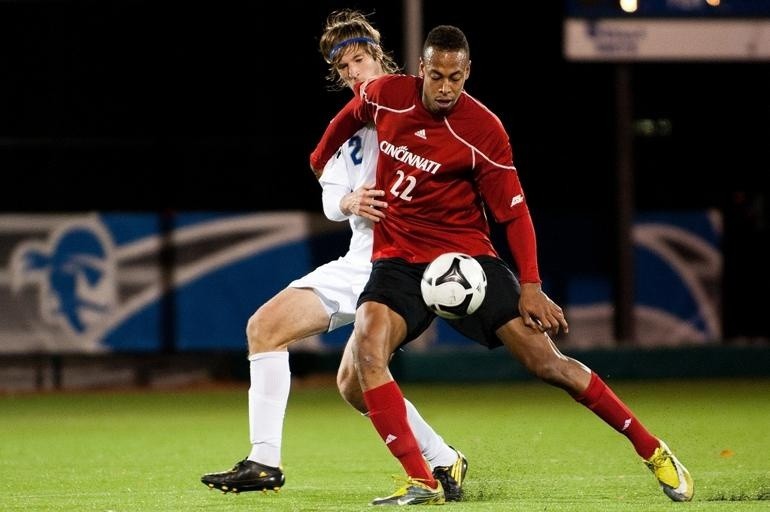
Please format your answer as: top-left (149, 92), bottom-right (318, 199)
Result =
top-left (371, 477), bottom-right (446, 507)
top-left (431, 446), bottom-right (469, 501)
top-left (638, 434), bottom-right (694, 503)
top-left (200, 456), bottom-right (285, 494)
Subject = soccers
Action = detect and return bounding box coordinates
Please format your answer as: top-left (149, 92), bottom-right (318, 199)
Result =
top-left (420, 253), bottom-right (487, 319)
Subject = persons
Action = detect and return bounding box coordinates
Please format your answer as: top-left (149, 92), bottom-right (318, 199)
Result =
top-left (310, 23), bottom-right (696, 505)
top-left (199, 9), bottom-right (468, 502)
